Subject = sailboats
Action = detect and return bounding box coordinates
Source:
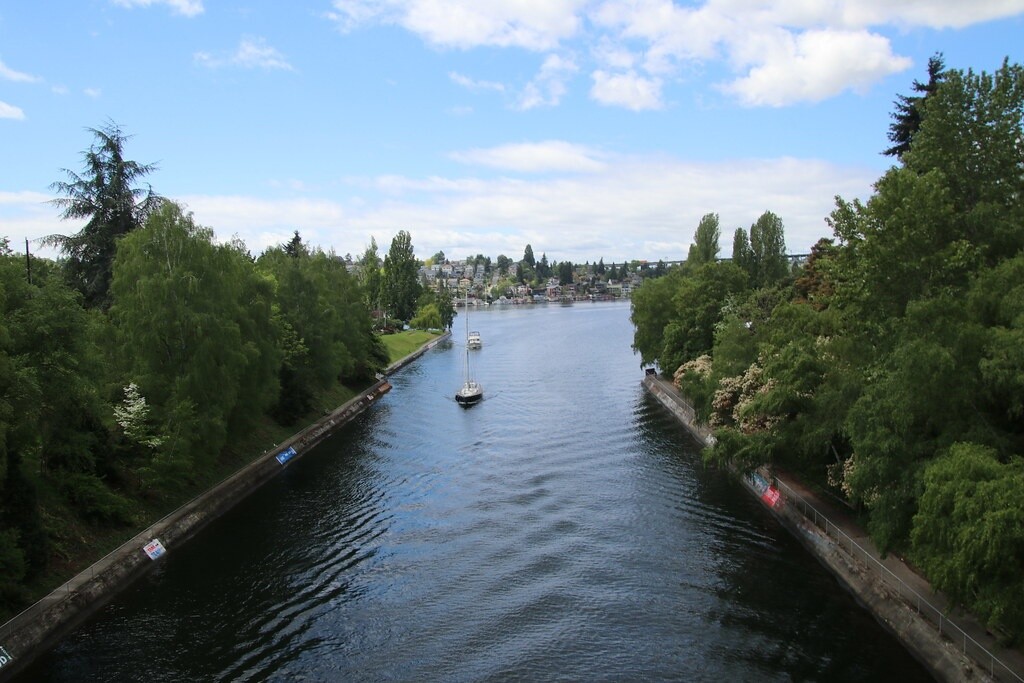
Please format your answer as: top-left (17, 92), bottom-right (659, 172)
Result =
top-left (465, 289), bottom-right (481, 350)
top-left (455, 346), bottom-right (483, 408)
